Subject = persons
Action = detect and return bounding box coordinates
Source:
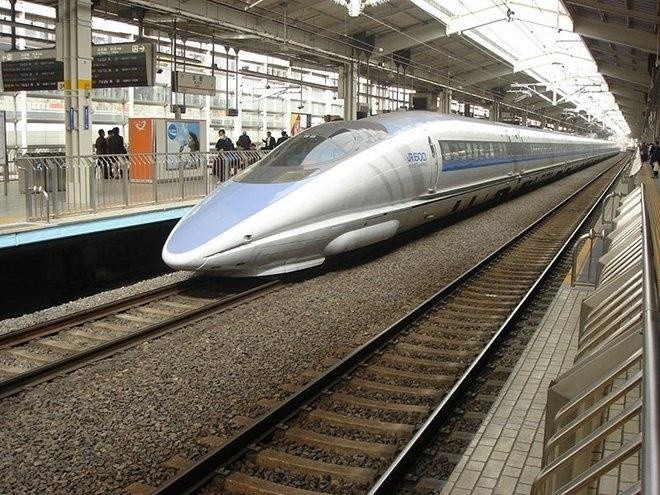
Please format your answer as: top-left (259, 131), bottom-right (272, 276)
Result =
top-left (639, 138), bottom-right (660, 179)
top-left (214, 129), bottom-right (234, 151)
top-left (261, 131), bottom-right (276, 150)
top-left (187, 131), bottom-right (200, 169)
top-left (94, 128), bottom-right (115, 179)
top-left (106, 129), bottom-right (120, 179)
top-left (277, 130), bottom-right (288, 146)
top-left (236, 130), bottom-right (251, 150)
top-left (109, 126), bottom-right (130, 181)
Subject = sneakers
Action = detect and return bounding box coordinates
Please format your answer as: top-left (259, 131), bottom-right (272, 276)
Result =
top-left (216, 180), bottom-right (224, 185)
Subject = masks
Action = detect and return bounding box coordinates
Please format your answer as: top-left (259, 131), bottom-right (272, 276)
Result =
top-left (219, 134), bottom-right (226, 139)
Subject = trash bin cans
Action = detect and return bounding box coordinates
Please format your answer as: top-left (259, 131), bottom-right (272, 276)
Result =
top-left (14, 151), bottom-right (66, 195)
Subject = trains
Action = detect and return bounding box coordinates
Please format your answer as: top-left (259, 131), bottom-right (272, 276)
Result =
top-left (160, 109), bottom-right (622, 280)
